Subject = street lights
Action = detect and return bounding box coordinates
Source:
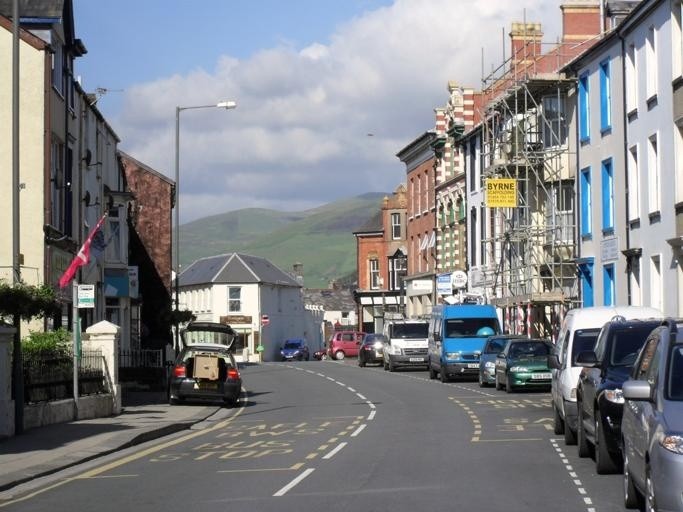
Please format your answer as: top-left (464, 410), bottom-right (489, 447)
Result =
top-left (175, 100), bottom-right (236, 357)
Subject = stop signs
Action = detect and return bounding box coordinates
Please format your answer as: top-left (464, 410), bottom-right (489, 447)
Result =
top-left (261, 315), bottom-right (269, 325)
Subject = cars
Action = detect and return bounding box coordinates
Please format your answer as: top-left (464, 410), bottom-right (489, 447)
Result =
top-left (167, 322), bottom-right (241, 405)
top-left (280, 337), bottom-right (309, 361)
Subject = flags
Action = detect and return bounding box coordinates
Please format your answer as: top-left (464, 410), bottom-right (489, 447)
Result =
top-left (59, 216), bottom-right (106, 288)
